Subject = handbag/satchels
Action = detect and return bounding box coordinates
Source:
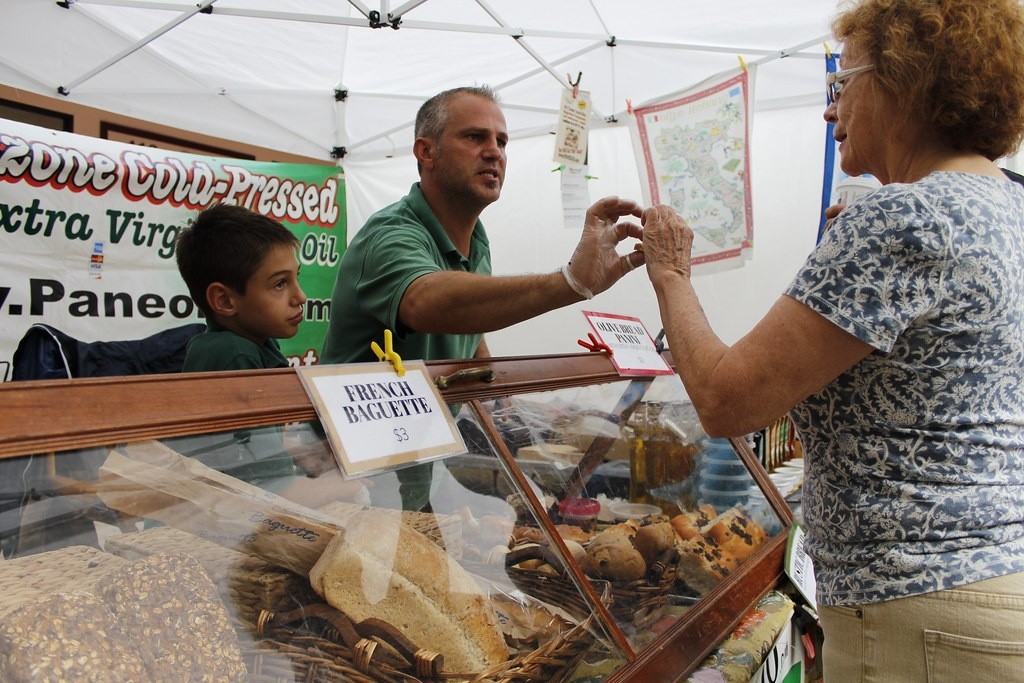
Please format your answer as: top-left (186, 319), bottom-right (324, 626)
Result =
top-left (11, 323), bottom-right (207, 382)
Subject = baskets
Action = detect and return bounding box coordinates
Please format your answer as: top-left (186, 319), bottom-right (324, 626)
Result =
top-left (0, 501), bottom-right (678, 683)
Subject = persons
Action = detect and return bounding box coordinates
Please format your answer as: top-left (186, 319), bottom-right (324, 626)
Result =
top-left (640, 0), bottom-right (1024, 683)
top-left (299, 87), bottom-right (646, 512)
top-left (165, 205), bottom-right (370, 514)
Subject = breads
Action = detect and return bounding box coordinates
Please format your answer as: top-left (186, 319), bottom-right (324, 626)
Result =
top-left (0, 412), bottom-right (767, 683)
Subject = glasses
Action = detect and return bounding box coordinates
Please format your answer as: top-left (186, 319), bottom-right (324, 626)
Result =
top-left (827, 64), bottom-right (877, 102)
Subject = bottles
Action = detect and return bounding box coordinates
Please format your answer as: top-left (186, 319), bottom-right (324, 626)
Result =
top-left (629, 402), bottom-right (702, 515)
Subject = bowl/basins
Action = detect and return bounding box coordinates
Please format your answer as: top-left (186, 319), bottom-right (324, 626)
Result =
top-left (611, 504), bottom-right (662, 524)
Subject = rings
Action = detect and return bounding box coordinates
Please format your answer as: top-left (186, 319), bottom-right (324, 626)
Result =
top-left (625, 256), bottom-right (635, 270)
